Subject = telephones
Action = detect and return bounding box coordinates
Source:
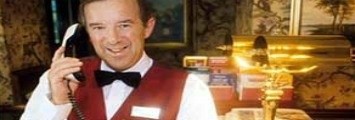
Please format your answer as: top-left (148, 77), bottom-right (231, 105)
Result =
top-left (59, 23), bottom-right (87, 84)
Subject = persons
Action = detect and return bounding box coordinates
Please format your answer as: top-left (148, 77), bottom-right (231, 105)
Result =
top-left (20, 0), bottom-right (218, 120)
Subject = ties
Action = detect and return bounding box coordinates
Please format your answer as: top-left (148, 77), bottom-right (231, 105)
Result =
top-left (94, 70), bottom-right (142, 88)
